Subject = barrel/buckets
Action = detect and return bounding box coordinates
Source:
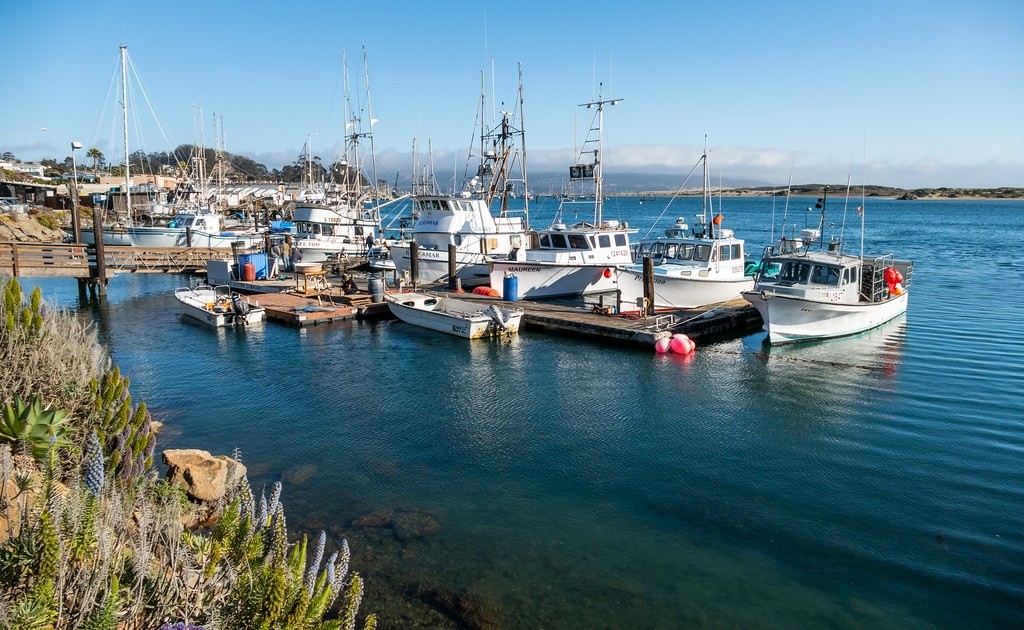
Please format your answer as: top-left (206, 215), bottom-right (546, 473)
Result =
top-left (243, 262), bottom-right (256, 281)
top-left (502, 276), bottom-right (519, 301)
top-left (367, 275), bottom-right (383, 295)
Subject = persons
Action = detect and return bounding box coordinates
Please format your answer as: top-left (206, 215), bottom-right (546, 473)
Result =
top-left (363, 232), bottom-right (377, 260)
top-left (571, 239), bottom-right (582, 249)
top-left (270, 241), bottom-right (281, 278)
top-left (340, 274), bottom-right (358, 296)
top-left (289, 246), bottom-right (303, 275)
top-left (286, 236), bottom-right (292, 254)
top-left (279, 240), bottom-right (291, 272)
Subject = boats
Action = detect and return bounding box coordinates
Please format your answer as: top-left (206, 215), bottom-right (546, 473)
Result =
top-left (383, 290), bottom-right (523, 339)
top-left (172, 284), bottom-right (266, 329)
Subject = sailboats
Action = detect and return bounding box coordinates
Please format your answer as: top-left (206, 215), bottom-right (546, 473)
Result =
top-left (617, 132), bottom-right (780, 317)
top-left (739, 183), bottom-right (912, 344)
top-left (62, 41), bottom-right (532, 287)
top-left (486, 78), bottom-right (640, 300)
